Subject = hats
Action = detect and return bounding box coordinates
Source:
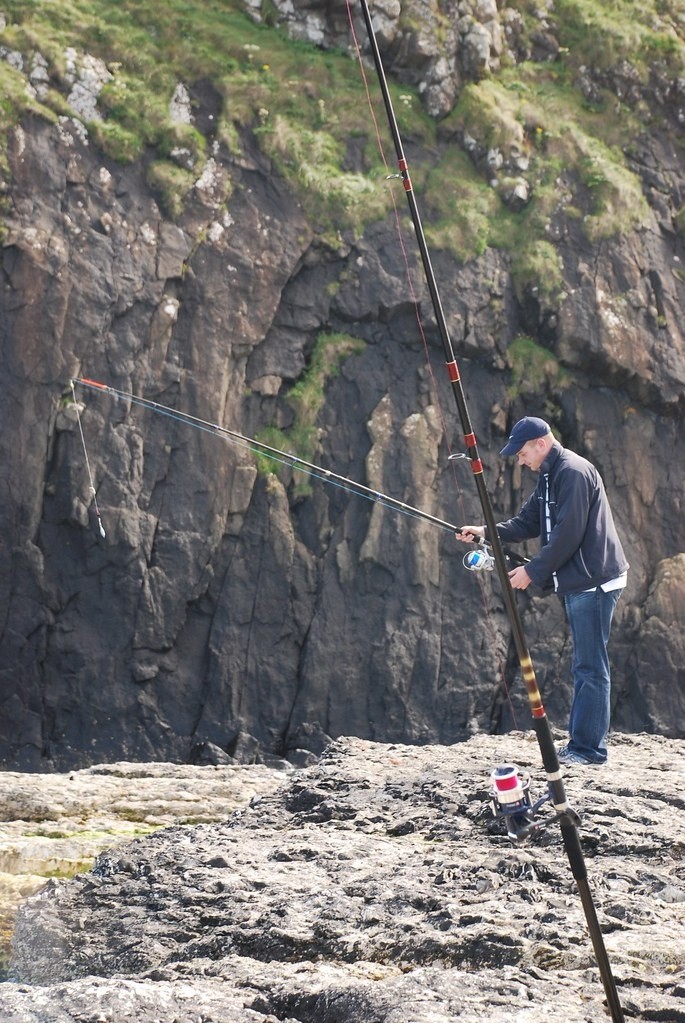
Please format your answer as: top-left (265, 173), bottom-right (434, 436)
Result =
top-left (499, 416), bottom-right (550, 456)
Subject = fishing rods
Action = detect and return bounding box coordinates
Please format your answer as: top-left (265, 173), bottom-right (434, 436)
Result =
top-left (361, 1), bottom-right (627, 1023)
top-left (71, 375), bottom-right (532, 571)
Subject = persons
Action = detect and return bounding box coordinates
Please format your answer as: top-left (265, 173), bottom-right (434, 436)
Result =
top-left (456, 416), bottom-right (630, 765)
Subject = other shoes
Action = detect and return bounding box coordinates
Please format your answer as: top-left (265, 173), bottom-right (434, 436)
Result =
top-left (556, 746), bottom-right (592, 765)
